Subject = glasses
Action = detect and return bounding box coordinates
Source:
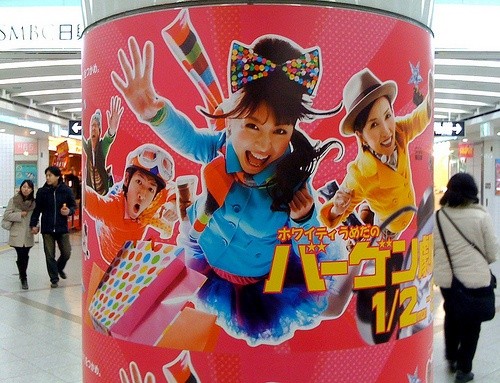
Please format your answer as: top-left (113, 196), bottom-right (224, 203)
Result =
top-left (140, 148), bottom-right (173, 176)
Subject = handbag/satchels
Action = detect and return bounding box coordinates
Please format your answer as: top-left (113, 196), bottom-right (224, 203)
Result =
top-left (449, 275), bottom-right (495, 322)
top-left (1, 197), bottom-right (16, 231)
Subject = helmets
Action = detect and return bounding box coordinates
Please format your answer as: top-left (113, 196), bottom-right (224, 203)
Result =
top-left (123, 144), bottom-right (175, 184)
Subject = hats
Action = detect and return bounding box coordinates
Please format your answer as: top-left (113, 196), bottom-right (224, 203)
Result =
top-left (339, 68), bottom-right (399, 138)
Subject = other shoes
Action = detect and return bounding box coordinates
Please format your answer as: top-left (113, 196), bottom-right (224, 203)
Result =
top-left (51, 278), bottom-right (59, 288)
top-left (58, 270), bottom-right (66, 279)
top-left (447, 358), bottom-right (457, 373)
top-left (22, 280), bottom-right (28, 289)
top-left (455, 372), bottom-right (474, 383)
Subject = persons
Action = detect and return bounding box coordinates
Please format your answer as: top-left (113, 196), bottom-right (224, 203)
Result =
top-left (82, 33), bottom-right (433, 382)
top-left (434, 173), bottom-right (498, 383)
top-left (3, 166), bottom-right (78, 288)
